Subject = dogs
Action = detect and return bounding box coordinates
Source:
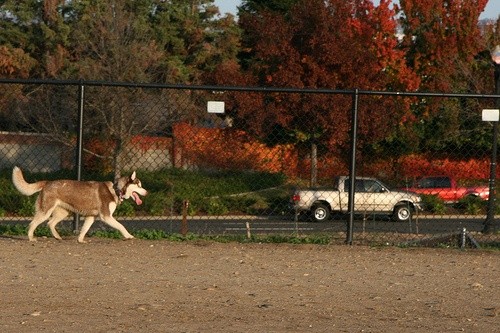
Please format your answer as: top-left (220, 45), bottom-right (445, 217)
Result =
top-left (12, 166), bottom-right (147, 244)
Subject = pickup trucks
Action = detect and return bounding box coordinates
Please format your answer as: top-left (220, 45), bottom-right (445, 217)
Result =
top-left (291, 176), bottom-right (422, 223)
top-left (403, 178), bottom-right (497, 207)
